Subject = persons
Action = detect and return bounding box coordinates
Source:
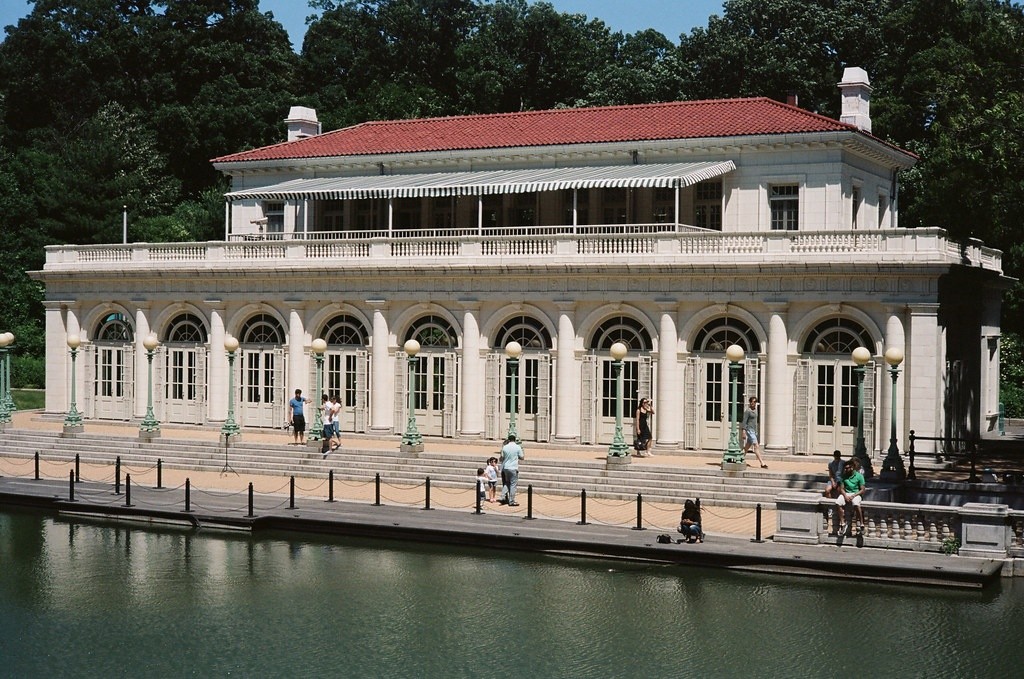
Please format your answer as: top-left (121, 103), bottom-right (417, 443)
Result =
top-left (742, 397), bottom-right (768, 468)
top-left (677, 500), bottom-right (700, 544)
top-left (485, 457), bottom-right (499, 503)
top-left (825, 449), bottom-right (845, 516)
top-left (477, 468), bottom-right (489, 509)
top-left (635, 398), bottom-right (655, 457)
top-left (835, 457), bottom-right (865, 534)
top-left (320, 395), bottom-right (342, 455)
top-left (498, 435), bottom-right (524, 506)
top-left (288, 389), bottom-right (311, 445)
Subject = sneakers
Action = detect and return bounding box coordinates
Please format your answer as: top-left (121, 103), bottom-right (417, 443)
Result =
top-left (860, 525), bottom-right (865, 536)
top-left (841, 523), bottom-right (848, 536)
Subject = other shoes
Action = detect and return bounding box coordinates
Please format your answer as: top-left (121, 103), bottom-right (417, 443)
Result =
top-left (637, 450), bottom-right (644, 457)
top-left (490, 498), bottom-right (496, 503)
top-left (761, 465), bottom-right (768, 468)
top-left (827, 509), bottom-right (834, 517)
top-left (510, 501), bottom-right (519, 506)
top-left (646, 450), bottom-right (654, 457)
top-left (323, 451), bottom-right (332, 456)
top-left (500, 500), bottom-right (508, 503)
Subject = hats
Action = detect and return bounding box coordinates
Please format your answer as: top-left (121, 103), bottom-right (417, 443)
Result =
top-left (834, 450), bottom-right (840, 457)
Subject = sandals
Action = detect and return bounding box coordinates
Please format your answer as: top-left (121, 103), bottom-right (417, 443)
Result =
top-left (686, 540), bottom-right (692, 543)
top-left (695, 538), bottom-right (701, 544)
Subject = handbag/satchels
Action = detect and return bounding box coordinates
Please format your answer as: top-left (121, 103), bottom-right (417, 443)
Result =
top-left (288, 426), bottom-right (295, 436)
top-left (633, 433), bottom-right (646, 450)
top-left (656, 534), bottom-right (674, 544)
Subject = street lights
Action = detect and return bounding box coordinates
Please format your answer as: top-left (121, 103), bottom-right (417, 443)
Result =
top-left (720, 343), bottom-right (749, 470)
top-left (880, 346), bottom-right (906, 481)
top-left (502, 341), bottom-right (525, 458)
top-left (218, 337), bottom-right (243, 443)
top-left (138, 335), bottom-right (162, 438)
top-left (849, 346), bottom-right (875, 480)
top-left (0, 333), bottom-right (14, 429)
top-left (401, 339), bottom-right (425, 452)
top-left (305, 337), bottom-right (328, 445)
top-left (62, 334), bottom-right (85, 433)
top-left (604, 342), bottom-right (632, 464)
top-left (2, 332), bottom-right (19, 417)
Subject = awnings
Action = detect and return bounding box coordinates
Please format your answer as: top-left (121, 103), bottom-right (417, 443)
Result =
top-left (224, 160), bottom-right (737, 233)
